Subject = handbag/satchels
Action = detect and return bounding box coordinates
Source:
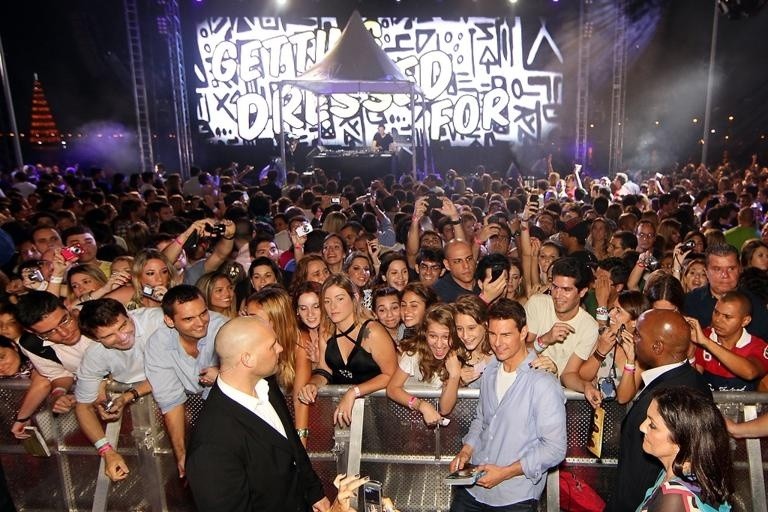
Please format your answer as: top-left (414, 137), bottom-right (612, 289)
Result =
top-left (596, 377), bottom-right (617, 401)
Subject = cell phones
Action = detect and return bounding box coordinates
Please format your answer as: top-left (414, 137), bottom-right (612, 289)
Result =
top-left (598, 326), bottom-right (618, 347)
top-left (367, 193), bottom-right (372, 204)
top-left (363, 480), bottom-right (383, 512)
top-left (372, 244), bottom-right (376, 252)
top-left (492, 265), bottom-right (504, 281)
top-left (331, 198), bottom-right (339, 203)
top-left (529, 195), bottom-right (539, 218)
top-left (142, 286), bottom-right (164, 303)
top-left (487, 216), bottom-right (499, 239)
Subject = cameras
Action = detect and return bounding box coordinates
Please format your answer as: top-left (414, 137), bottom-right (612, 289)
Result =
top-left (204, 223), bottom-right (226, 234)
top-left (643, 256), bottom-right (659, 271)
top-left (29, 269), bottom-right (45, 282)
top-left (424, 194), bottom-right (442, 209)
top-left (61, 243), bottom-right (85, 261)
top-left (681, 240), bottom-right (696, 253)
top-left (295, 223), bottom-right (314, 237)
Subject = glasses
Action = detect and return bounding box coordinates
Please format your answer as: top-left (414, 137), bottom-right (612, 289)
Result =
top-left (639, 233), bottom-right (653, 238)
top-left (34, 316), bottom-right (73, 337)
top-left (609, 244), bottom-right (621, 250)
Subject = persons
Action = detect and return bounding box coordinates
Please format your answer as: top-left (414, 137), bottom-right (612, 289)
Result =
top-left (370, 124), bottom-right (396, 152)
top-left (0, 138), bottom-right (767, 512)
